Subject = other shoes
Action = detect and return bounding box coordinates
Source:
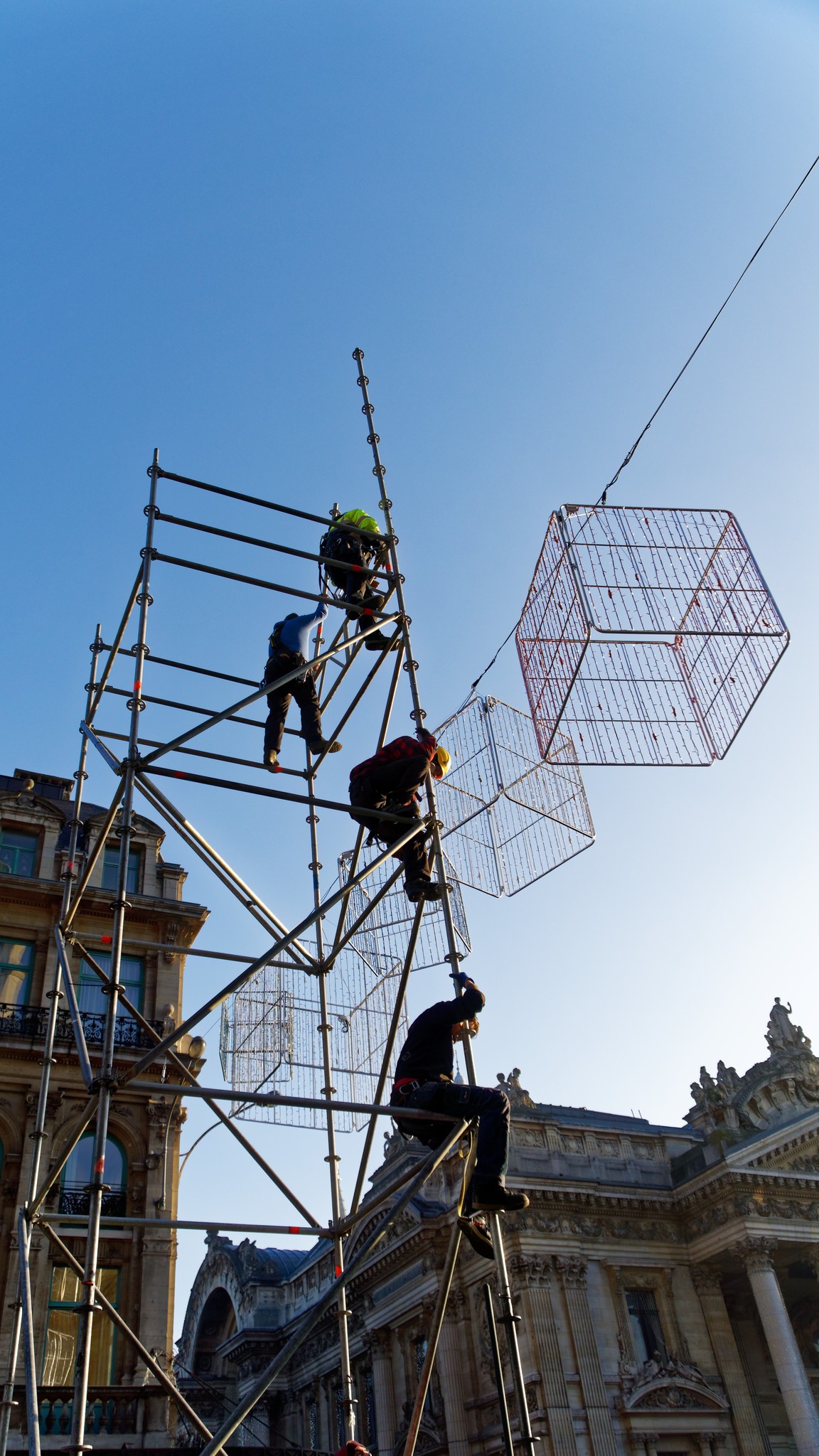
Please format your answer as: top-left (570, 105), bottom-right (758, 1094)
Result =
top-left (309, 740), bottom-right (343, 755)
top-left (382, 802), bottom-right (421, 817)
top-left (262, 750), bottom-right (280, 774)
top-left (365, 636), bottom-right (402, 651)
top-left (347, 595), bottom-right (384, 621)
top-left (407, 878), bottom-right (453, 902)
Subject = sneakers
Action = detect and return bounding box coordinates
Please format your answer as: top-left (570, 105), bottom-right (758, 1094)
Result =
top-left (474, 1187), bottom-right (530, 1210)
top-left (457, 1216), bottom-right (494, 1259)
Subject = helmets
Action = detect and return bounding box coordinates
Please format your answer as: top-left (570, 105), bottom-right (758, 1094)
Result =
top-left (433, 746), bottom-right (451, 779)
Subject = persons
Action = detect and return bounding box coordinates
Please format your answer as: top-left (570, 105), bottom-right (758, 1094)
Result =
top-left (264, 593), bottom-right (342, 774)
top-left (495, 1069), bottom-right (537, 1108)
top-left (324, 508), bottom-right (401, 652)
top-left (389, 970), bottom-right (530, 1259)
top-left (689, 1059), bottom-right (741, 1102)
top-left (632, 1350), bottom-right (715, 1394)
top-left (767, 997), bottom-right (812, 1052)
top-left (347, 728), bottom-right (454, 903)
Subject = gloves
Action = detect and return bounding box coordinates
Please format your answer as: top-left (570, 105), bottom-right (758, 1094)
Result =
top-left (449, 972), bottom-right (475, 989)
top-left (415, 727), bottom-right (431, 739)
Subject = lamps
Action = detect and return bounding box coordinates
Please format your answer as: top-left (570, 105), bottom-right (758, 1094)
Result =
top-left (150, 1036), bottom-right (204, 1211)
